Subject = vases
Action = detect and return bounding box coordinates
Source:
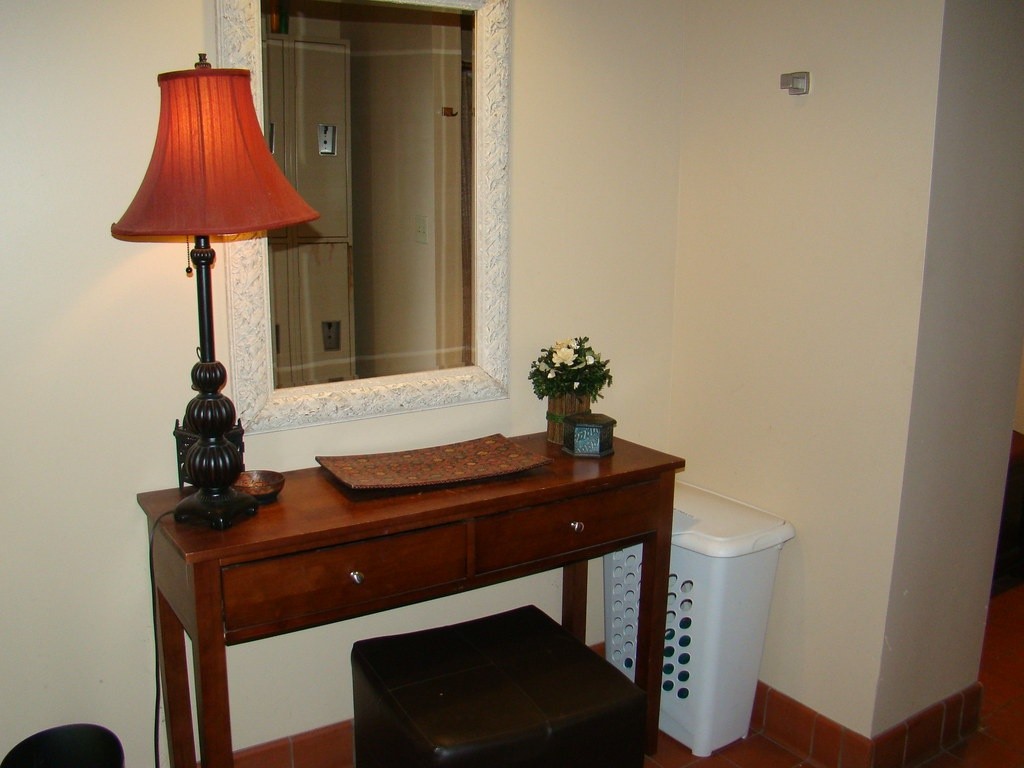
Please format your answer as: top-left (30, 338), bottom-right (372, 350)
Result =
top-left (542, 394), bottom-right (591, 447)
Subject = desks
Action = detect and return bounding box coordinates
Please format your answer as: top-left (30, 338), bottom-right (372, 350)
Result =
top-left (137, 432), bottom-right (684, 768)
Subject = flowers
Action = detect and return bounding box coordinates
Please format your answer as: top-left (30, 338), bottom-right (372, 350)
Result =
top-left (529, 336), bottom-right (613, 403)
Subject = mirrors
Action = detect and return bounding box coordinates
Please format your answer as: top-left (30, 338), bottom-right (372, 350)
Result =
top-left (214, 0), bottom-right (512, 431)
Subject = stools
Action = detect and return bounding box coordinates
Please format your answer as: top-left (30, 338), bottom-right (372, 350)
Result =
top-left (351, 605), bottom-right (644, 768)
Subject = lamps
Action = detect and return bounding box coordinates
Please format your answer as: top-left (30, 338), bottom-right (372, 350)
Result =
top-left (111, 53), bottom-right (321, 488)
